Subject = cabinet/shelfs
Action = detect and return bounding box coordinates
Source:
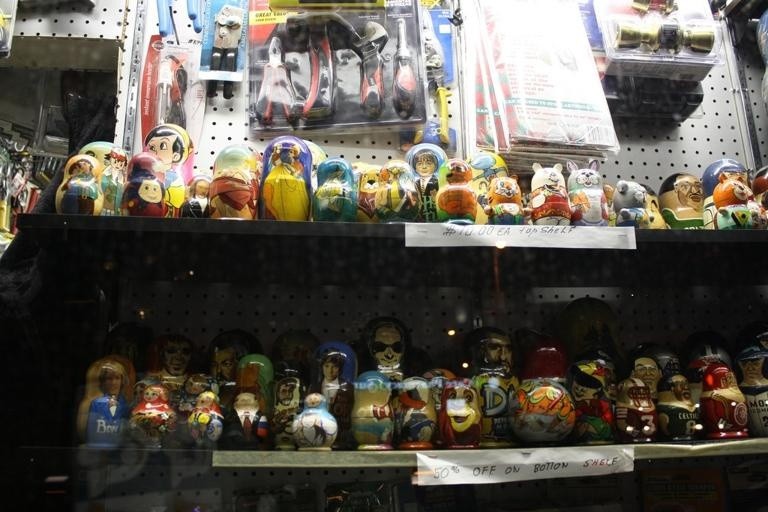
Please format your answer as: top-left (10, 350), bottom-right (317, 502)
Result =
top-left (15, 213), bottom-right (768, 468)
